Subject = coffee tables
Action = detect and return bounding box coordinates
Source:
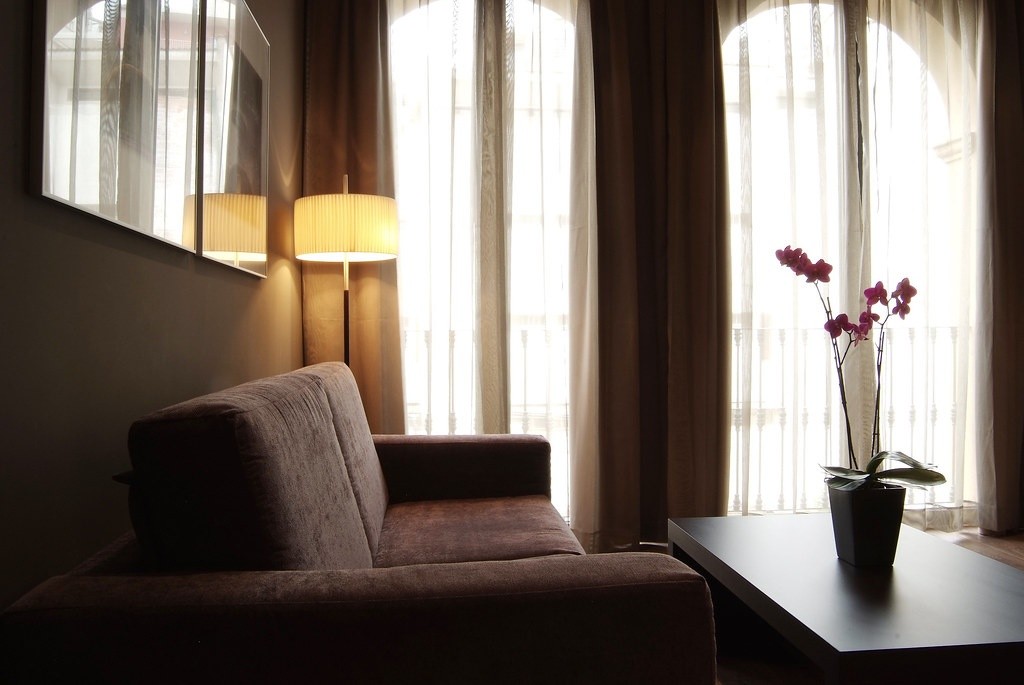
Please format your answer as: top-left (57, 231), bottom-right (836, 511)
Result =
top-left (667, 514), bottom-right (1023, 685)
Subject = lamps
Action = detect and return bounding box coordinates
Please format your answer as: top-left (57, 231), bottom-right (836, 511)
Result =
top-left (293, 174), bottom-right (400, 368)
top-left (183, 191), bottom-right (268, 267)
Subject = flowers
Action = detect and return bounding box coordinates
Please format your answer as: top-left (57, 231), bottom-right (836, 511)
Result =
top-left (775, 244), bottom-right (948, 492)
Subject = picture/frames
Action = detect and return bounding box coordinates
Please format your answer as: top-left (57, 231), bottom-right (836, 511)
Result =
top-left (196, 0), bottom-right (271, 278)
top-left (41, 0), bottom-right (201, 254)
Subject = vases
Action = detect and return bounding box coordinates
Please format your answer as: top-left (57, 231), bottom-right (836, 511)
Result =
top-left (828, 483), bottom-right (906, 568)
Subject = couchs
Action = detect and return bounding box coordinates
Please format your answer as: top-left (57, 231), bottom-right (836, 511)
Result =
top-left (0, 361), bottom-right (718, 684)
